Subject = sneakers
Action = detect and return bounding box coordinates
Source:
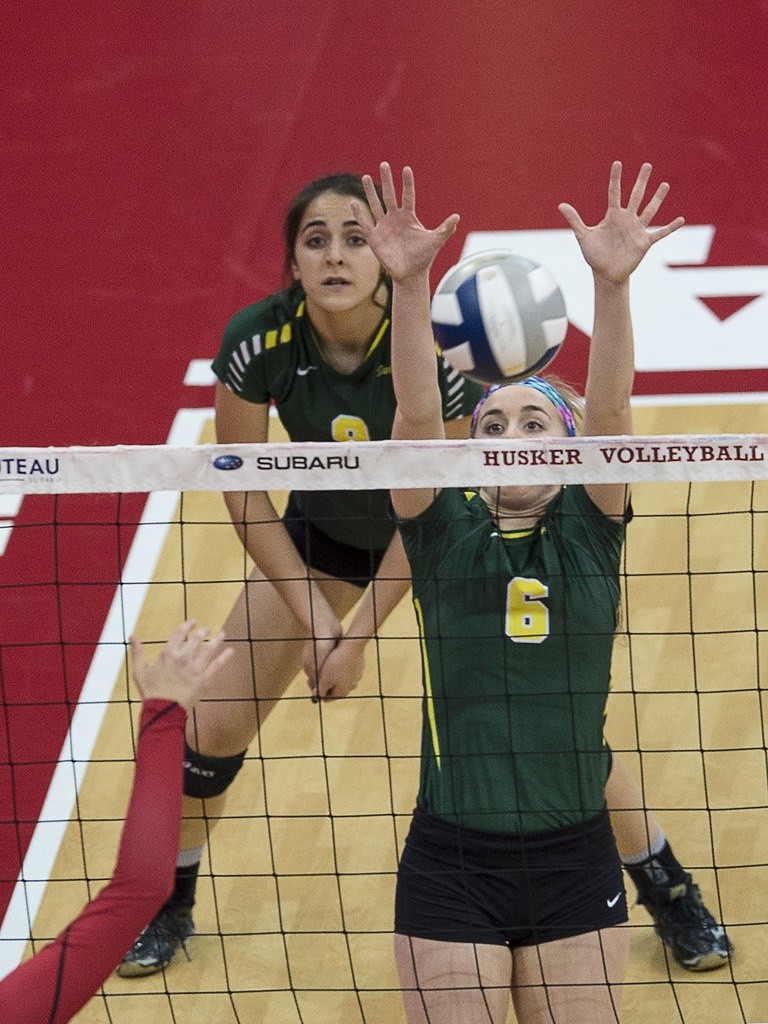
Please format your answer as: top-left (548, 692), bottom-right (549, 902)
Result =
top-left (639, 874), bottom-right (735, 970)
top-left (116, 872), bottom-right (195, 977)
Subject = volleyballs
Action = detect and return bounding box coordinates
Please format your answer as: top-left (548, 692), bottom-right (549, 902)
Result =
top-left (430, 248), bottom-right (568, 385)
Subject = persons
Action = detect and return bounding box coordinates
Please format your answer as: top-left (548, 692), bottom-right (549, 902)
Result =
top-left (116, 173), bottom-right (735, 977)
top-left (0, 619), bottom-right (234, 1024)
top-left (349, 161), bottom-right (686, 1024)
